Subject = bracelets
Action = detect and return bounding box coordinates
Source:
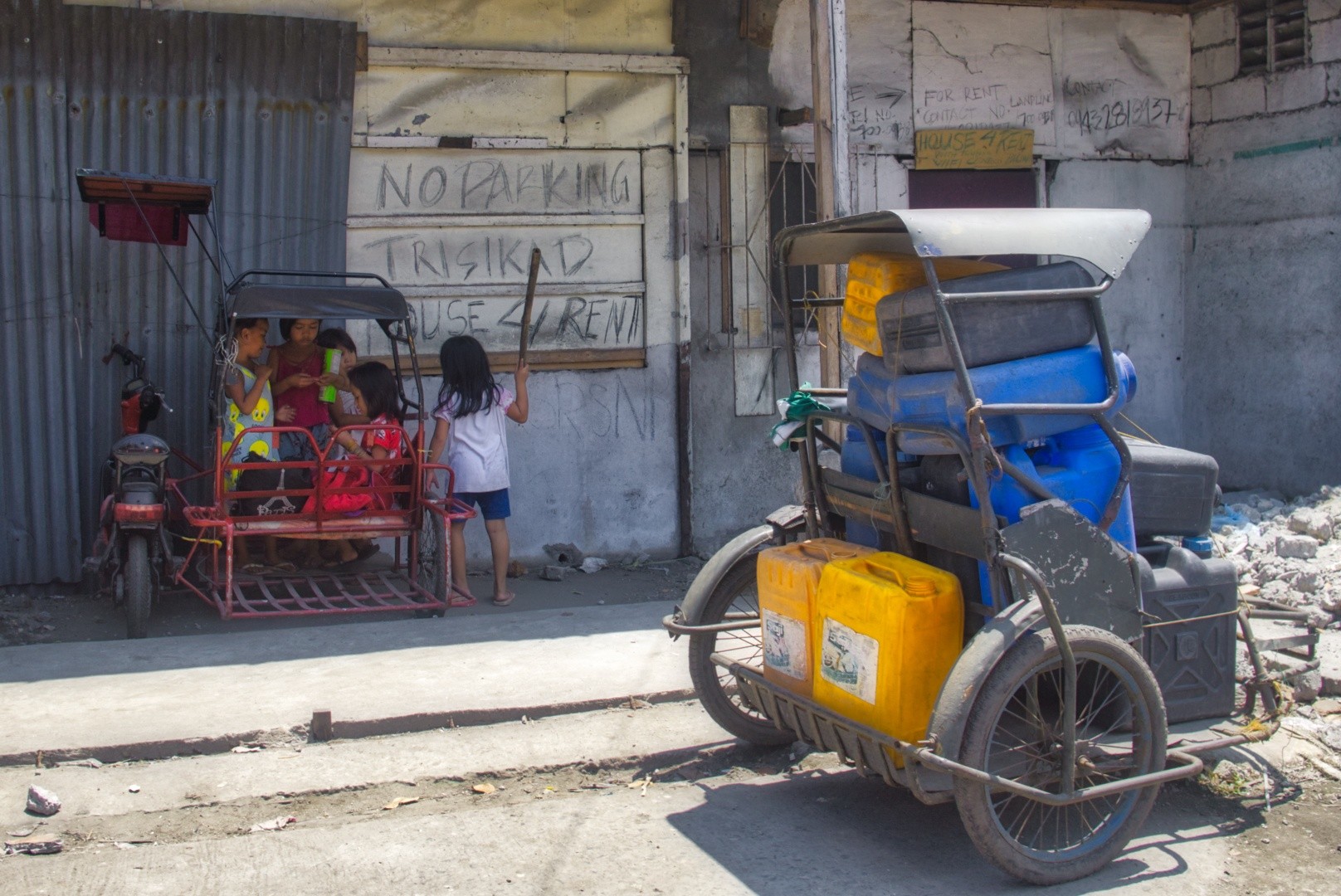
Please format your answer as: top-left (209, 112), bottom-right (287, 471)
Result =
top-left (349, 444), bottom-right (369, 459)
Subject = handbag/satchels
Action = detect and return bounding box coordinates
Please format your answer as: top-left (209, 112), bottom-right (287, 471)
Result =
top-left (300, 454), bottom-right (377, 514)
top-left (232, 450), bottom-right (315, 515)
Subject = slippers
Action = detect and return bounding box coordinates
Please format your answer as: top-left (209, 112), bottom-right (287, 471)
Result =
top-left (237, 564), bottom-right (273, 576)
top-left (265, 562), bottom-right (304, 575)
top-left (320, 553), bottom-right (363, 571)
top-left (493, 590), bottom-right (515, 606)
top-left (357, 545), bottom-right (380, 560)
top-left (449, 591), bottom-right (469, 601)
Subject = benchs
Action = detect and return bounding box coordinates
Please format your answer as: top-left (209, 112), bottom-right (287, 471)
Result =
top-left (222, 423), bottom-right (418, 539)
top-left (802, 410), bottom-right (1145, 673)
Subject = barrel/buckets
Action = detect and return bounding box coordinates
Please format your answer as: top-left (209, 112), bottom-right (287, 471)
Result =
top-left (756, 251), bottom-right (1237, 768)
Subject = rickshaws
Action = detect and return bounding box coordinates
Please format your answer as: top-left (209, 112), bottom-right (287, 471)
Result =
top-left (664, 205), bottom-right (1320, 887)
top-left (74, 165), bottom-right (476, 633)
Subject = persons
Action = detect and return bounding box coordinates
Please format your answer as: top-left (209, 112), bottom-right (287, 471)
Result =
top-left (314, 326), bottom-right (380, 560)
top-left (267, 318), bottom-right (331, 565)
top-left (220, 319), bottom-right (297, 577)
top-left (299, 361), bottom-right (404, 569)
top-left (425, 335), bottom-right (530, 605)
